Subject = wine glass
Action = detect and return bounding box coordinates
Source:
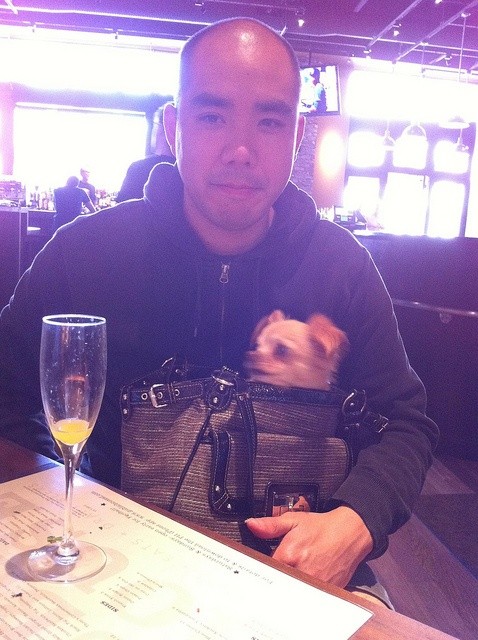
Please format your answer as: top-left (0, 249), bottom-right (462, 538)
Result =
top-left (26, 314), bottom-right (107, 582)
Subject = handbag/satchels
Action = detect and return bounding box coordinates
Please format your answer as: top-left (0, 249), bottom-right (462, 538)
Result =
top-left (116, 350), bottom-right (393, 559)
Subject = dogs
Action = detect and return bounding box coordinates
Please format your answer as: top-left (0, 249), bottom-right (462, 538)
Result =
top-left (241, 308), bottom-right (348, 393)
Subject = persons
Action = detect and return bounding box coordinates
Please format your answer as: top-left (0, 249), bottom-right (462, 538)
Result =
top-left (116, 102), bottom-right (176, 205)
top-left (302, 68), bottom-right (327, 113)
top-left (0, 16), bottom-right (440, 613)
top-left (53, 176), bottom-right (96, 234)
top-left (78, 167), bottom-right (95, 207)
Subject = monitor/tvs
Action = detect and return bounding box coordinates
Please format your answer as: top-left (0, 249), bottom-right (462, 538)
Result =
top-left (294, 63), bottom-right (343, 116)
